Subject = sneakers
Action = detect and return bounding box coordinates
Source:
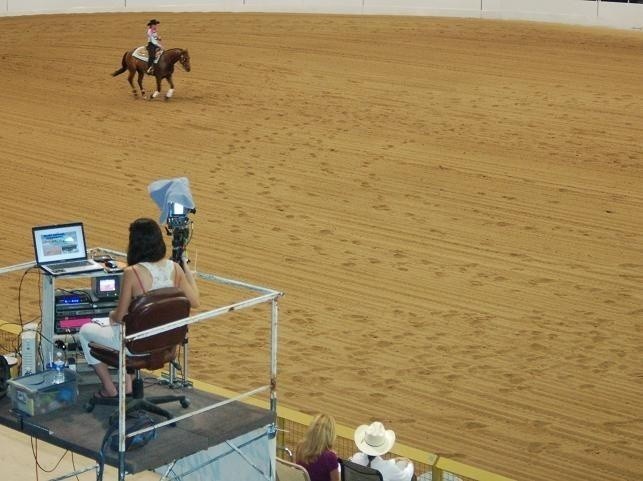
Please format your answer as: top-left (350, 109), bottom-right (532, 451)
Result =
top-left (147, 66), bottom-right (153, 73)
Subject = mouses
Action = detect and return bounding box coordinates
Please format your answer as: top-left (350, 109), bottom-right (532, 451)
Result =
top-left (105, 260), bottom-right (117, 268)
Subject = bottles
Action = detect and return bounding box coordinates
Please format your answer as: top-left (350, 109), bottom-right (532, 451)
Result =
top-left (41, 343), bottom-right (67, 386)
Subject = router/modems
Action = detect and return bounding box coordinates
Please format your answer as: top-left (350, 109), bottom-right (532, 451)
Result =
top-left (22, 323), bottom-right (38, 376)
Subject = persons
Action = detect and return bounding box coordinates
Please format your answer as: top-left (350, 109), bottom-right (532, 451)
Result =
top-left (145, 19), bottom-right (163, 74)
top-left (292, 413), bottom-right (340, 480)
top-left (348, 421), bottom-right (417, 481)
top-left (78, 216), bottom-right (202, 405)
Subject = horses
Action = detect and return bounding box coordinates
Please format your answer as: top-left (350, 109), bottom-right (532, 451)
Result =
top-left (110, 47), bottom-right (191, 101)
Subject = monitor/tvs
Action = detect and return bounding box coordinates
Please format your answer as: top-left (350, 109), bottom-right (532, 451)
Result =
top-left (91, 276), bottom-right (120, 302)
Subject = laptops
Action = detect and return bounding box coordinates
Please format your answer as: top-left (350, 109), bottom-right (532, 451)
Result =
top-left (32, 222), bottom-right (103, 277)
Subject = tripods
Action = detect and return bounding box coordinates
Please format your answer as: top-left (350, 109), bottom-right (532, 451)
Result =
top-left (168, 228), bottom-right (188, 388)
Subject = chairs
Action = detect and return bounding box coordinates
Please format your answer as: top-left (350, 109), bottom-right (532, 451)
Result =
top-left (337, 457), bottom-right (383, 481)
top-left (83, 287), bottom-right (191, 428)
top-left (276, 456), bottom-right (311, 481)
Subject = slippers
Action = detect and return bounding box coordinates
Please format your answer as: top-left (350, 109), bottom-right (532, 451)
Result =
top-left (92, 389), bottom-right (119, 399)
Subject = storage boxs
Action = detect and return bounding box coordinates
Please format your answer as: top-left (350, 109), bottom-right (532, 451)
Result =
top-left (5, 366), bottom-right (79, 417)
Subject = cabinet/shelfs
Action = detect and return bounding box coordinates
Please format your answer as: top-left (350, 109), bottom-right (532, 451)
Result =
top-left (41, 262), bottom-right (128, 385)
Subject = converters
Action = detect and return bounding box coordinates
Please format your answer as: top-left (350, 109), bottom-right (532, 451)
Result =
top-left (55, 340), bottom-right (66, 350)
top-left (68, 343), bottom-right (77, 351)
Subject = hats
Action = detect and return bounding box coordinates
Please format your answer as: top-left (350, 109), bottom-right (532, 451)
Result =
top-left (354, 421), bottom-right (396, 457)
top-left (148, 20), bottom-right (159, 25)
